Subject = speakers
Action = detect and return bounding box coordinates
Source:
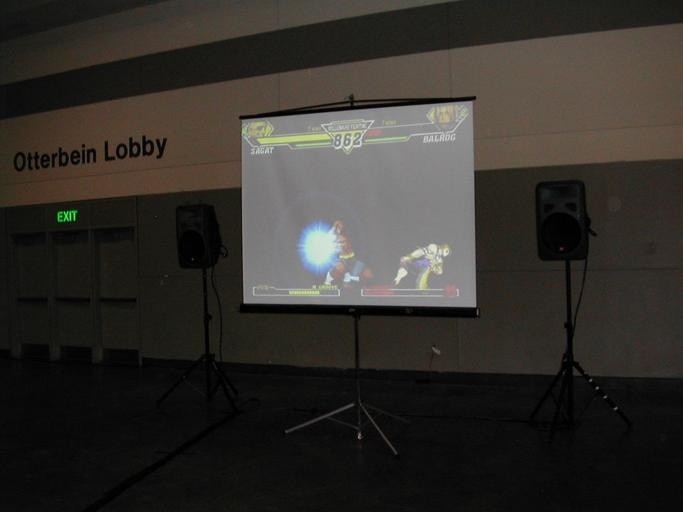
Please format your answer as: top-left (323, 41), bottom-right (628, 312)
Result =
top-left (535, 179), bottom-right (589, 261)
top-left (177, 204), bottom-right (221, 269)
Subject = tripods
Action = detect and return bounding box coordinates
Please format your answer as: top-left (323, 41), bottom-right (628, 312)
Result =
top-left (283, 314), bottom-right (408, 456)
top-left (156, 269), bottom-right (241, 430)
top-left (521, 262), bottom-right (633, 441)
